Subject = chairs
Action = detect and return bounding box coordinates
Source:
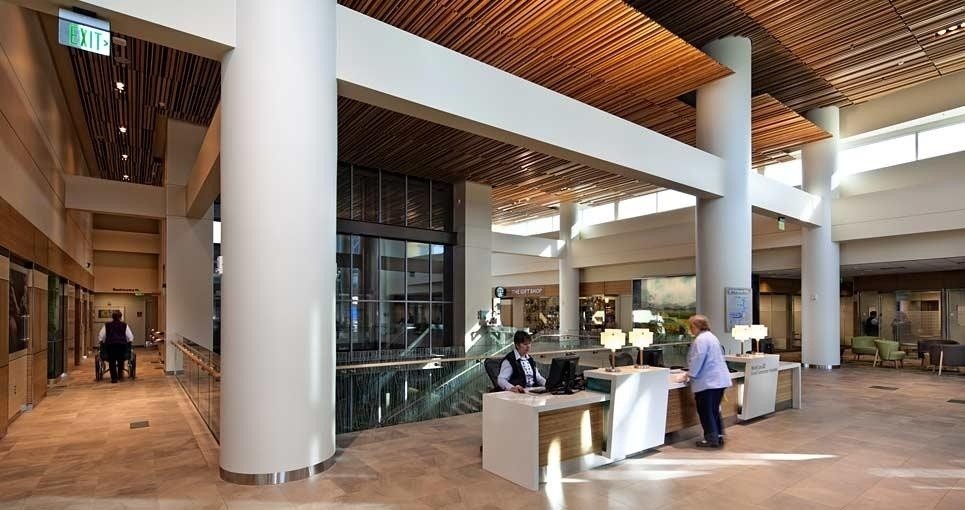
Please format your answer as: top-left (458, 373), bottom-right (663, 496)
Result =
top-left (872, 340), bottom-right (906, 370)
top-left (479, 358), bottom-right (505, 452)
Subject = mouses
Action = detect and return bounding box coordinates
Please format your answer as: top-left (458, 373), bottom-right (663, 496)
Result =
top-left (518, 389), bottom-right (525, 393)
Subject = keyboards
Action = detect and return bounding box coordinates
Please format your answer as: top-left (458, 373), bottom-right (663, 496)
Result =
top-left (529, 388), bottom-right (561, 393)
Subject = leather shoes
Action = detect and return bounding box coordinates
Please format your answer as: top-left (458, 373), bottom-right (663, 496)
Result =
top-left (696, 436), bottom-right (723, 448)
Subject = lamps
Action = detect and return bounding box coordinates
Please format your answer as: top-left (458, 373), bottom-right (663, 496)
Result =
top-left (629, 328), bottom-right (654, 369)
top-left (600, 329), bottom-right (626, 372)
top-left (752, 325), bottom-right (769, 356)
top-left (731, 325), bottom-right (750, 358)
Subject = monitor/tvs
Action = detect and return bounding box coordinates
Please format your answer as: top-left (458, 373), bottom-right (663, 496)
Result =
top-left (547, 356), bottom-right (580, 395)
top-left (637, 350), bottom-right (662, 367)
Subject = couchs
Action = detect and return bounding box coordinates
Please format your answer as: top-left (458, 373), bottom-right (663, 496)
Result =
top-left (851, 336), bottom-right (885, 365)
top-left (917, 339), bottom-right (965, 377)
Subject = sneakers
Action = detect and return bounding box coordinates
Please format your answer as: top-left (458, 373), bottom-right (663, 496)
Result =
top-left (111, 380), bottom-right (118, 383)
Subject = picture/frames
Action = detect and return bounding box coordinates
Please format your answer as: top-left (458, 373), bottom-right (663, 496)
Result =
top-left (724, 287), bottom-right (753, 333)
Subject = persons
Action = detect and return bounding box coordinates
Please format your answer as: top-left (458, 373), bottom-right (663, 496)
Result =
top-left (676, 314), bottom-right (734, 450)
top-left (499, 329), bottom-right (549, 394)
top-left (892, 311), bottom-right (900, 343)
top-left (866, 310), bottom-right (884, 337)
top-left (97, 309), bottom-right (133, 383)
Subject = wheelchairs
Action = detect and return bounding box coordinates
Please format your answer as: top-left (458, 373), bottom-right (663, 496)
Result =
top-left (94, 340), bottom-right (136, 382)
